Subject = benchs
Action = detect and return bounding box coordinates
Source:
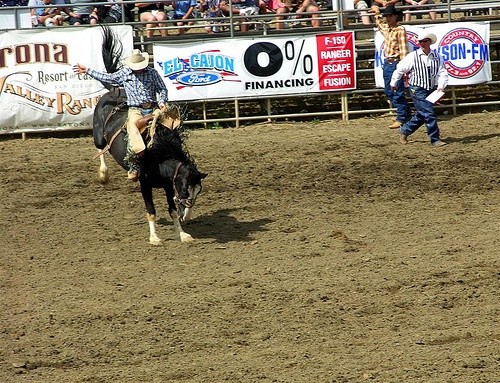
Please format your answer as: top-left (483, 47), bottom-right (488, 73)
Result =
top-left (33, 0), bottom-right (500, 43)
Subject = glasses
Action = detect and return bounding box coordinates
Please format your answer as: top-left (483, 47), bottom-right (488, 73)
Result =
top-left (419, 40), bottom-right (429, 43)
top-left (385, 15), bottom-right (392, 17)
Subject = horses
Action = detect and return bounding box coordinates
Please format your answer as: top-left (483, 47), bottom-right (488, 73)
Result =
top-left (93, 20), bottom-right (208, 245)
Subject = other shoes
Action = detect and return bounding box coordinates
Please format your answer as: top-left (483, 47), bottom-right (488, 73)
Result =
top-left (432, 140), bottom-right (447, 147)
top-left (127, 163), bottom-right (139, 179)
top-left (399, 128), bottom-right (407, 144)
top-left (389, 122), bottom-right (403, 128)
top-left (392, 117), bottom-right (397, 121)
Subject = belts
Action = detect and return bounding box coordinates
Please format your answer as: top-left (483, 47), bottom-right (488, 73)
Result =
top-left (139, 102), bottom-right (155, 109)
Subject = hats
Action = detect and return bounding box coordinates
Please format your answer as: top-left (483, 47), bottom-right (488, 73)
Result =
top-left (417, 32), bottom-right (437, 45)
top-left (125, 49), bottom-right (149, 71)
top-left (381, 5), bottom-right (397, 14)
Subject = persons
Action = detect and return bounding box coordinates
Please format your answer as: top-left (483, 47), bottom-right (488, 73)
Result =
top-left (389, 33), bottom-right (448, 147)
top-left (27, 0), bottom-right (439, 38)
top-left (380, 5), bottom-right (410, 130)
top-left (73, 49), bottom-right (168, 180)
